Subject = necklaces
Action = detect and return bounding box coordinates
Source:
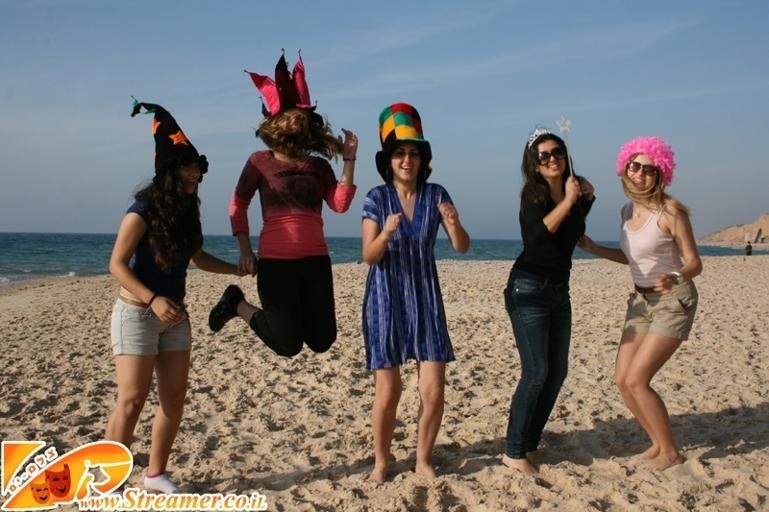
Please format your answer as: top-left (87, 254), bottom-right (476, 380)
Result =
top-left (634, 211), bottom-right (643, 219)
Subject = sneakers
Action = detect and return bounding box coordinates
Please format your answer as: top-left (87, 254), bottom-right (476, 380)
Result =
top-left (209, 285), bottom-right (244, 330)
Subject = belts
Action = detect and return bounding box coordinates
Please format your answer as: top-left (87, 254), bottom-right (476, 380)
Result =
top-left (118, 293), bottom-right (188, 309)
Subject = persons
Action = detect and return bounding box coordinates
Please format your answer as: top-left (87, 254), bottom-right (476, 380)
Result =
top-left (208, 50), bottom-right (359, 359)
top-left (500, 127), bottom-right (597, 474)
top-left (362, 102), bottom-right (470, 485)
top-left (745, 241), bottom-right (752, 255)
top-left (576, 137), bottom-right (704, 472)
top-left (91, 98), bottom-right (252, 511)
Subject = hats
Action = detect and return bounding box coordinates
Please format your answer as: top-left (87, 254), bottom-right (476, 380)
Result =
top-left (376, 103), bottom-right (432, 184)
top-left (617, 137), bottom-right (675, 188)
top-left (244, 48), bottom-right (325, 136)
top-left (131, 102), bottom-right (208, 184)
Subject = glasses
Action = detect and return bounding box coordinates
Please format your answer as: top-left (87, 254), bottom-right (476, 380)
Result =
top-left (626, 161), bottom-right (659, 178)
top-left (536, 146), bottom-right (565, 166)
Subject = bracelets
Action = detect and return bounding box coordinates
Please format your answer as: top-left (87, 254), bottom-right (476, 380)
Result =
top-left (672, 271), bottom-right (684, 284)
top-left (342, 157), bottom-right (356, 161)
top-left (147, 294), bottom-right (157, 306)
